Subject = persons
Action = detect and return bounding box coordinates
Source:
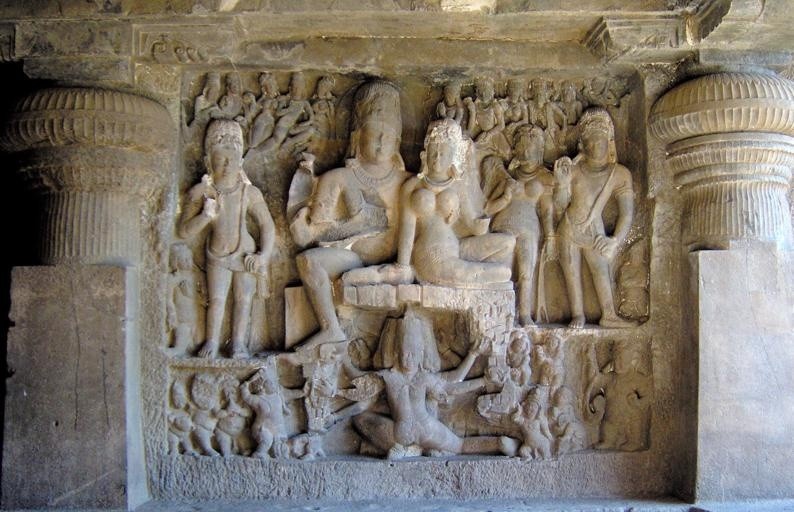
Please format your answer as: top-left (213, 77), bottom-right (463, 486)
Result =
top-left (167, 70), bottom-right (655, 464)
